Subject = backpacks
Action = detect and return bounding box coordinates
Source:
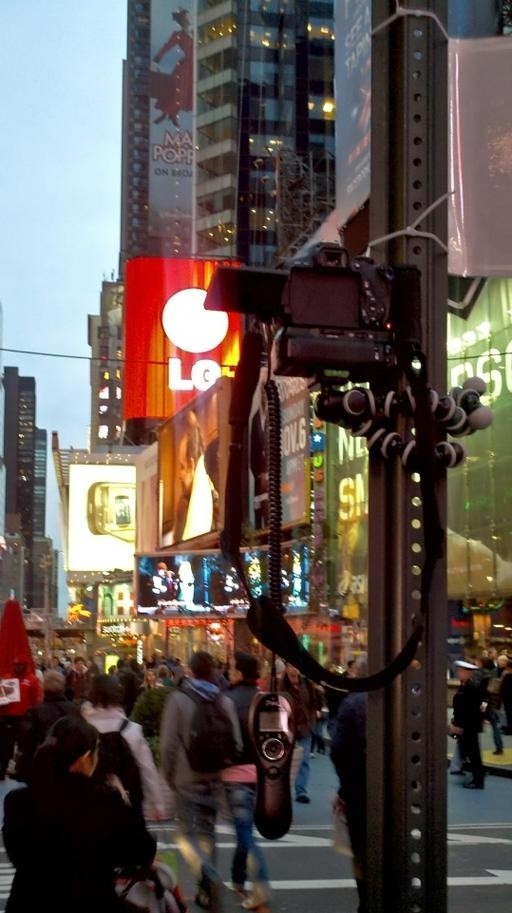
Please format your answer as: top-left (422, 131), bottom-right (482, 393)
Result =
top-left (90, 718), bottom-right (145, 808)
top-left (177, 688), bottom-right (231, 774)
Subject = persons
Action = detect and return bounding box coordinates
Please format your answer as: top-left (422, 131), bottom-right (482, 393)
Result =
top-left (2, 643), bottom-right (371, 913)
top-left (146, 558), bottom-right (181, 608)
top-left (448, 643), bottom-right (511, 790)
top-left (147, 7), bottom-right (194, 127)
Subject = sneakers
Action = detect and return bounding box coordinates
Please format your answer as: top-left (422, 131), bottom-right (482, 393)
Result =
top-left (493, 749), bottom-right (504, 755)
top-left (194, 864), bottom-right (222, 912)
top-left (462, 780), bottom-right (485, 790)
top-left (241, 893), bottom-right (273, 910)
top-left (310, 749), bottom-right (326, 758)
top-left (450, 770), bottom-right (467, 776)
top-left (295, 794), bottom-right (310, 804)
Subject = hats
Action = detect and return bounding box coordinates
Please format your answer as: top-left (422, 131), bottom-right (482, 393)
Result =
top-left (454, 660), bottom-right (478, 671)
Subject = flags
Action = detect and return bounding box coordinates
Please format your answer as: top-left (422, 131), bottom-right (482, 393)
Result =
top-left (0, 587), bottom-right (39, 716)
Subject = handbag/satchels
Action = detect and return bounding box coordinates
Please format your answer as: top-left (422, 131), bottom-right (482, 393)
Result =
top-left (113, 859), bottom-right (189, 912)
